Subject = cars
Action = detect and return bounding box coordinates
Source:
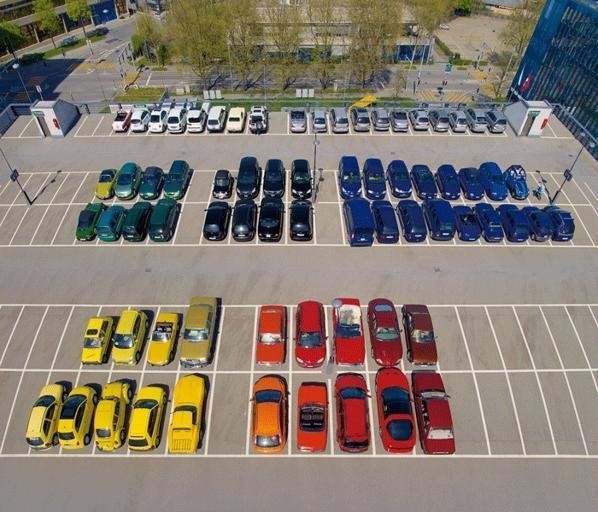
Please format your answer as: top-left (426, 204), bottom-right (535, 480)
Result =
top-left (20, 51), bottom-right (42, 65)
top-left (439, 23), bottom-right (450, 31)
top-left (58, 34), bottom-right (80, 47)
top-left (85, 26), bottom-right (109, 38)
top-left (150, 1), bottom-right (165, 16)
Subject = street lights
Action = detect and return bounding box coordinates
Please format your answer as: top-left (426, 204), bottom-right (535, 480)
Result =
top-left (13, 62), bottom-right (34, 104)
top-left (102, 8), bottom-right (112, 31)
